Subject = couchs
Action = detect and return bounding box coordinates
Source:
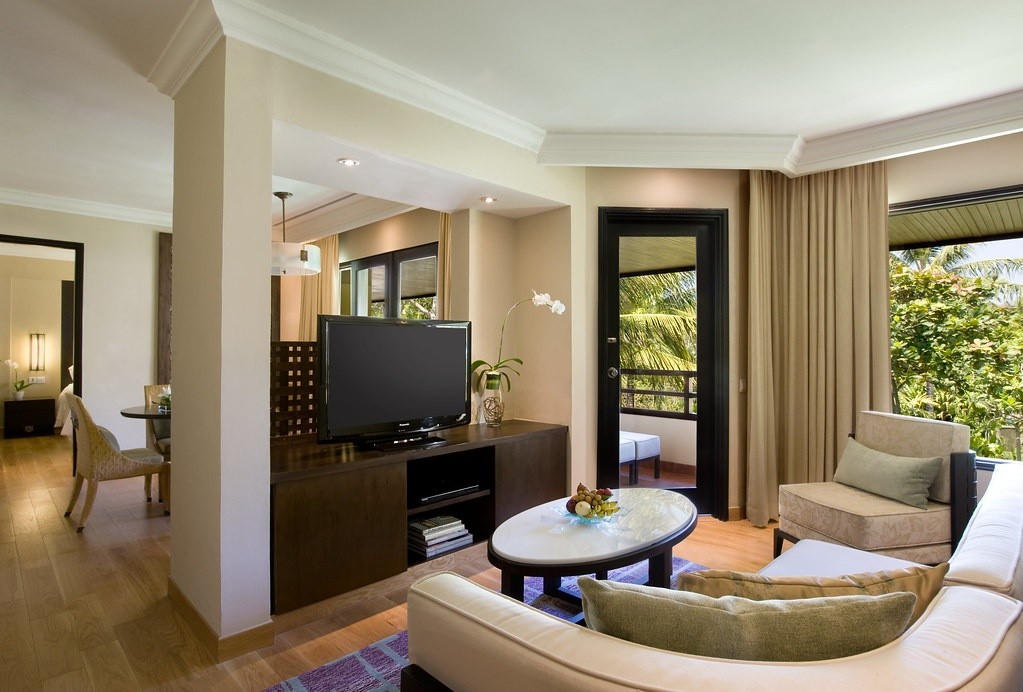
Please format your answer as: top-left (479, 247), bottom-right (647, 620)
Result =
top-left (772, 409), bottom-right (971, 566)
top-left (397, 460), bottom-right (1023, 691)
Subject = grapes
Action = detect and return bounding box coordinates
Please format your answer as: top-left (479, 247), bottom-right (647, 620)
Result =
top-left (570, 489), bottom-right (603, 507)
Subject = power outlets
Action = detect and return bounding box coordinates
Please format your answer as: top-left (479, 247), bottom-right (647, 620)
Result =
top-left (28, 377), bottom-right (45, 383)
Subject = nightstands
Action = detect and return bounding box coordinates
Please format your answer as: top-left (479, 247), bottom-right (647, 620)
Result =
top-left (4, 398), bottom-right (55, 439)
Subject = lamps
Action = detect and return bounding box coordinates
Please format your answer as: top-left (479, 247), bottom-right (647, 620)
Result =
top-left (480, 196), bottom-right (496, 203)
top-left (339, 158), bottom-right (360, 166)
top-left (269, 192), bottom-right (322, 277)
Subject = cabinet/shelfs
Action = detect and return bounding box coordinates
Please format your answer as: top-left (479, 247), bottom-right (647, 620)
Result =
top-left (270, 417), bottom-right (570, 616)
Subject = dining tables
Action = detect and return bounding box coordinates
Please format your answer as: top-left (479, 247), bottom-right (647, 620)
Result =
top-left (119, 404), bottom-right (172, 421)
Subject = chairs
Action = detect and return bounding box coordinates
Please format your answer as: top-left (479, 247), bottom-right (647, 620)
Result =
top-left (63, 393), bottom-right (173, 532)
top-left (143, 384), bottom-right (172, 504)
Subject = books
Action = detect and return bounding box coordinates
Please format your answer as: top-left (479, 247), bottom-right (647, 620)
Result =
top-left (408, 512), bottom-right (472, 557)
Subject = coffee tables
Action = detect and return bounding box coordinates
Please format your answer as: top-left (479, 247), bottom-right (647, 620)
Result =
top-left (487, 487), bottom-right (698, 630)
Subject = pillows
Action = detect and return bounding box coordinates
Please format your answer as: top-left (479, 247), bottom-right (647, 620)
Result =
top-left (575, 575), bottom-right (917, 663)
top-left (675, 563), bottom-right (952, 633)
top-left (832, 436), bottom-right (943, 510)
top-left (68, 364), bottom-right (73, 382)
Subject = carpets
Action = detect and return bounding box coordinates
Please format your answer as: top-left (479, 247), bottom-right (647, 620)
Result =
top-left (259, 555), bottom-right (711, 692)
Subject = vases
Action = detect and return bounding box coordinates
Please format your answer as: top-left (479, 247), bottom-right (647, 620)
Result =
top-left (13, 391), bottom-right (25, 401)
top-left (482, 373), bottom-right (505, 428)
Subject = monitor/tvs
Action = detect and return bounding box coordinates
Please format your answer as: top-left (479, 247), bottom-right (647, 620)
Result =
top-left (317, 313), bottom-right (473, 443)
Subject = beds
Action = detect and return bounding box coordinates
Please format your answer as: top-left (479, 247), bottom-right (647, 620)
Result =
top-left (54, 383), bottom-right (73, 440)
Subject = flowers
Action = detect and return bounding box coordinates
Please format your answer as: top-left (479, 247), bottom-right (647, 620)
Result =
top-left (470, 289), bottom-right (565, 422)
top-left (1, 359), bottom-right (34, 393)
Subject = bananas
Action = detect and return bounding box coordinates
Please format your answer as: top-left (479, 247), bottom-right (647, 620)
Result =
top-left (584, 500), bottom-right (620, 518)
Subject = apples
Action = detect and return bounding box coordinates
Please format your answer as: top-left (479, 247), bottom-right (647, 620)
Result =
top-left (565, 500), bottom-right (578, 514)
